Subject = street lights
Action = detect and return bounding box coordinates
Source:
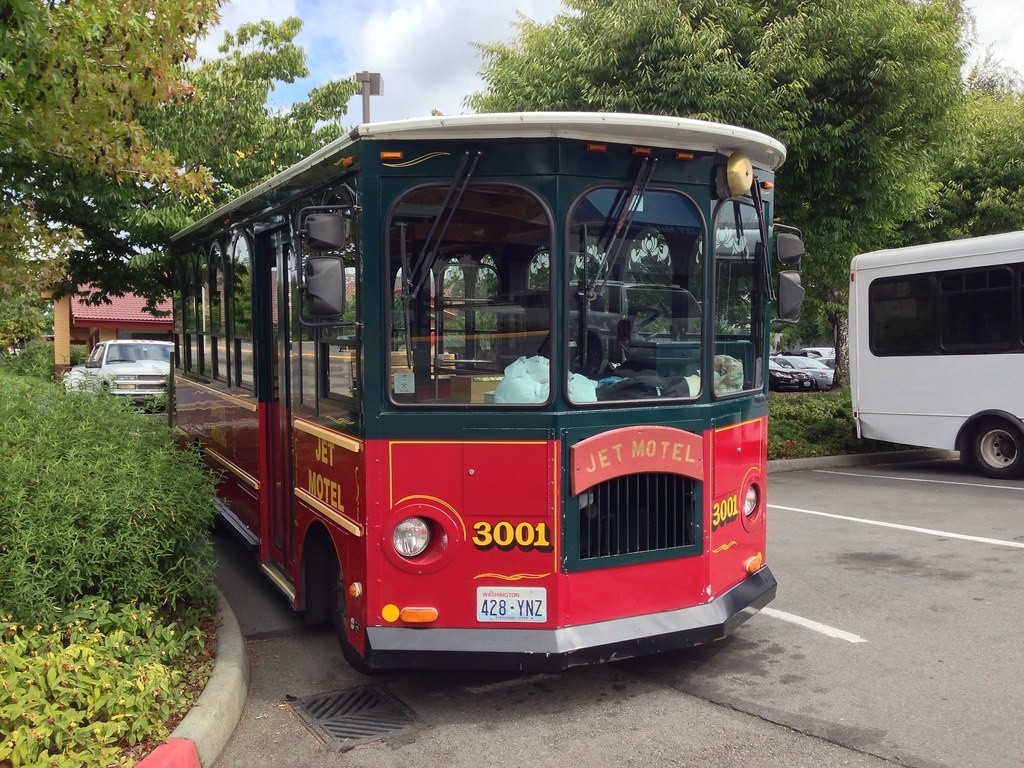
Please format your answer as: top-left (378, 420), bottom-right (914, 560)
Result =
top-left (354, 72), bottom-right (385, 124)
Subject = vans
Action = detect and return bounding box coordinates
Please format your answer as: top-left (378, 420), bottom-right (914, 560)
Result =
top-left (566, 279), bottom-right (705, 344)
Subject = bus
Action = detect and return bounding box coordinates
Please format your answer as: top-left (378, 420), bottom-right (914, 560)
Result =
top-left (167, 112), bottom-right (807, 680)
top-left (848, 230), bottom-right (1024, 480)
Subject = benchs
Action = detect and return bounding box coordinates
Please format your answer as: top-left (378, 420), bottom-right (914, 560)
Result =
top-left (345, 351), bottom-right (458, 391)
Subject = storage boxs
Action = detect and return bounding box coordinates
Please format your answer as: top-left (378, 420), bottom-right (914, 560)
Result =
top-left (450, 373), bottom-right (506, 403)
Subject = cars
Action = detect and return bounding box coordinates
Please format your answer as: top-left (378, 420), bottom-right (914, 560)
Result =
top-left (337, 335), bottom-right (355, 352)
top-left (85, 340), bottom-right (174, 403)
top-left (802, 347), bottom-right (836, 370)
top-left (786, 355), bottom-right (836, 391)
top-left (768, 357), bottom-right (818, 392)
